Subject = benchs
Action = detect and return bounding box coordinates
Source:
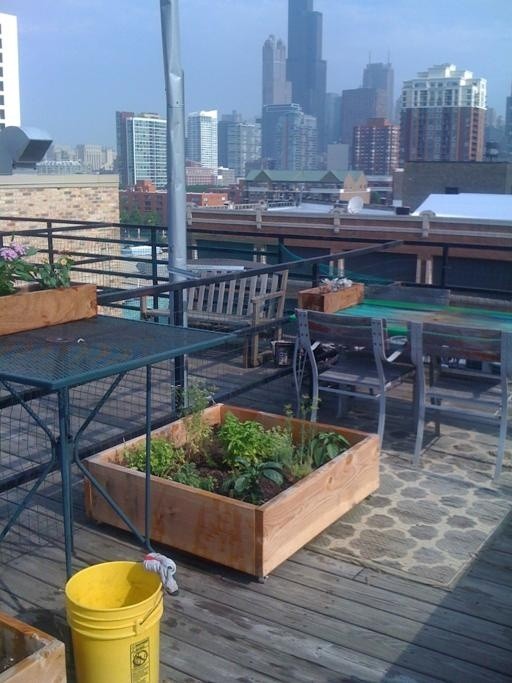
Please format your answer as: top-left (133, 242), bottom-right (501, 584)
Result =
top-left (134, 262), bottom-right (290, 370)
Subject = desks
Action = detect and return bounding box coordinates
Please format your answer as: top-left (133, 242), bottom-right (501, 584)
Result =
top-left (0, 314), bottom-right (240, 577)
top-left (288, 299), bottom-right (512, 447)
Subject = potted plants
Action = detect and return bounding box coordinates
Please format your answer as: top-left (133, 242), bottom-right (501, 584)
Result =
top-left (83, 371), bottom-right (384, 586)
top-left (0, 242), bottom-right (98, 334)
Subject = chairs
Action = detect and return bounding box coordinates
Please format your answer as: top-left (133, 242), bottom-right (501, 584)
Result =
top-left (290, 301), bottom-right (443, 450)
top-left (405, 317), bottom-right (511, 480)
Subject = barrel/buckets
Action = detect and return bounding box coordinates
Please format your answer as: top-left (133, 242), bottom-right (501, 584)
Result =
top-left (65, 562), bottom-right (164, 683)
